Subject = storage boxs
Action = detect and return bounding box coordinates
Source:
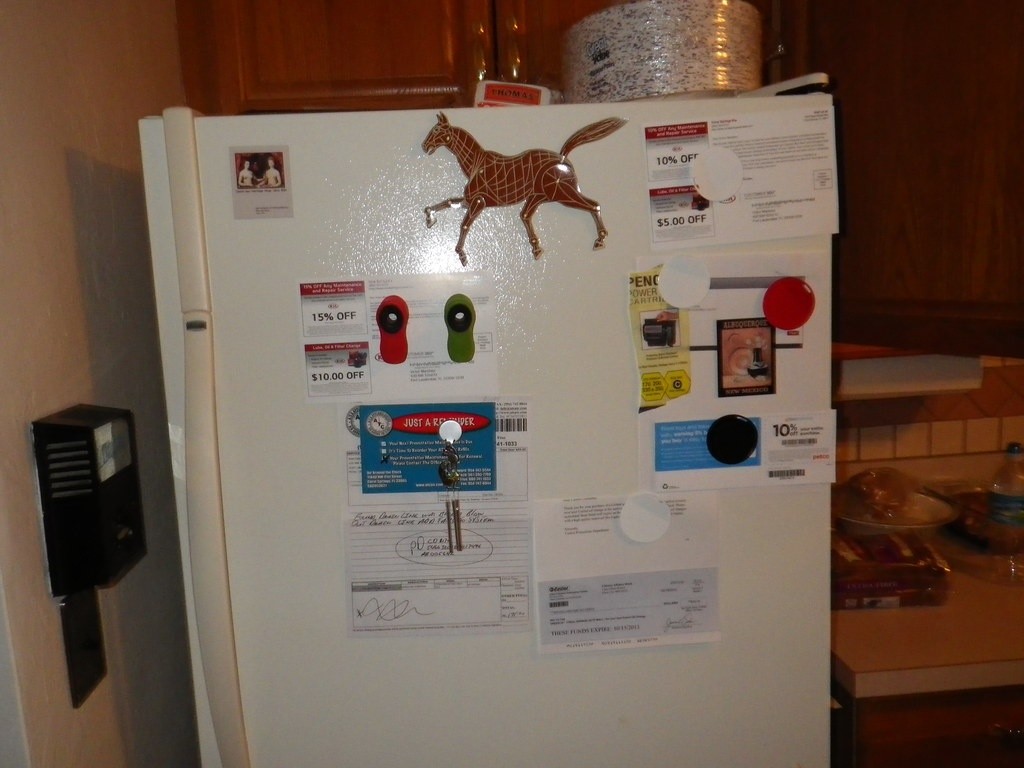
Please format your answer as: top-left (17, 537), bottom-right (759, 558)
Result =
top-left (473, 81), bottom-right (550, 108)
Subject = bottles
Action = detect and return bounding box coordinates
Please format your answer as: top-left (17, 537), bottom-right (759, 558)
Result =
top-left (989, 442), bottom-right (1024, 527)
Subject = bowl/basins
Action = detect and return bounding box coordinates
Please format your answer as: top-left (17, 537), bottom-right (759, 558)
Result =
top-left (834, 488), bottom-right (958, 541)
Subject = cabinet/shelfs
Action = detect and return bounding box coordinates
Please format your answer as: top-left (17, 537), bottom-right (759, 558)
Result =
top-left (173, 0), bottom-right (1024, 428)
top-left (831, 682), bottom-right (1024, 768)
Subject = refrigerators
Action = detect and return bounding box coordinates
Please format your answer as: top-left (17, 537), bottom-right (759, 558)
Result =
top-left (140, 72), bottom-right (845, 768)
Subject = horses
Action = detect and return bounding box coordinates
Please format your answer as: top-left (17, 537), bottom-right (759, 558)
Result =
top-left (420, 109), bottom-right (629, 267)
